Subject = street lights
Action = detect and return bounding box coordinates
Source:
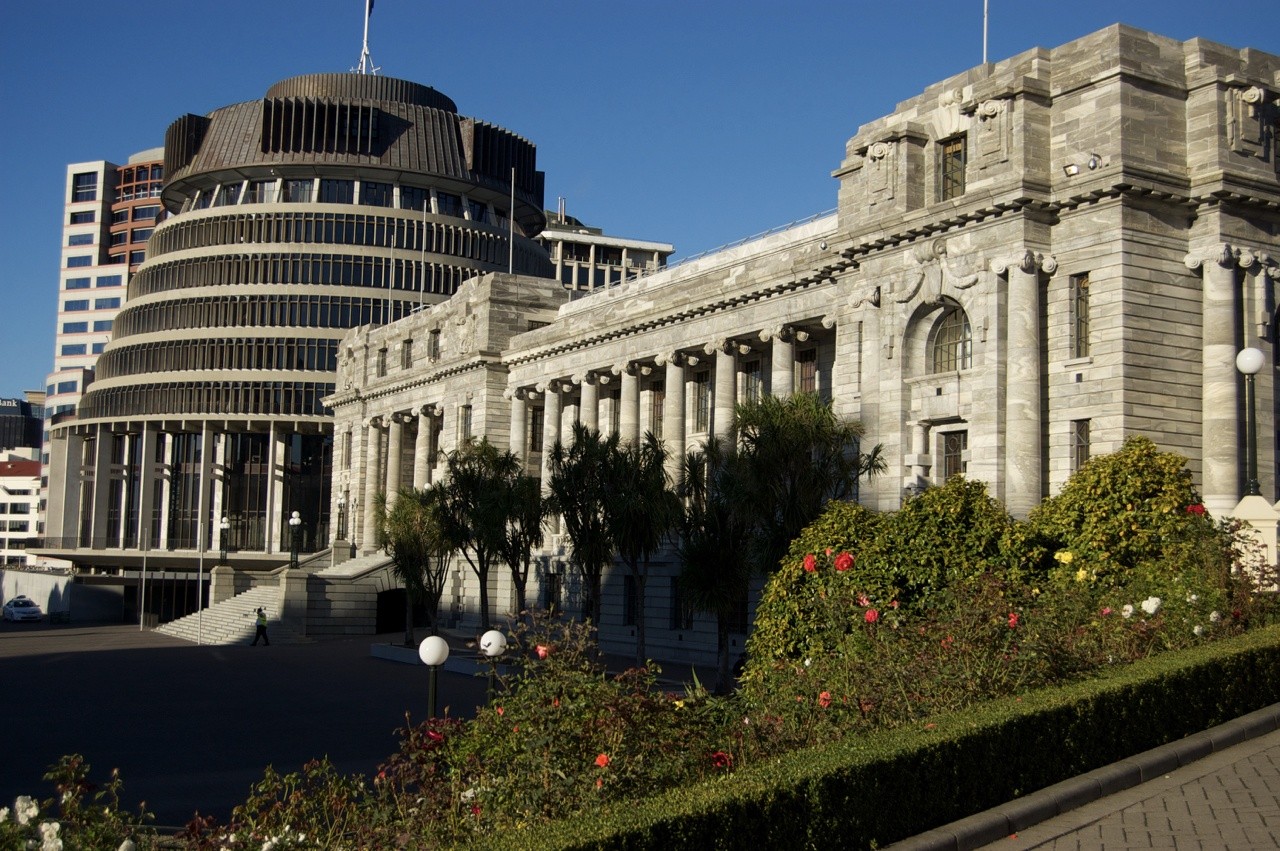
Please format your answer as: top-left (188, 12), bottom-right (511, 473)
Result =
top-left (479, 630), bottom-right (507, 711)
top-left (1236, 347), bottom-right (1266, 496)
top-left (219, 516), bottom-right (230, 566)
top-left (419, 636), bottom-right (449, 721)
top-left (288, 510), bottom-right (301, 569)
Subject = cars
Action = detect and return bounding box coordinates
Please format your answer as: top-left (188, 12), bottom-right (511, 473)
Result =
top-left (2, 598), bottom-right (43, 623)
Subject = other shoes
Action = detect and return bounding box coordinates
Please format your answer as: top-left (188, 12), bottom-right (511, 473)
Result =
top-left (250, 643), bottom-right (256, 647)
top-left (264, 643), bottom-right (269, 646)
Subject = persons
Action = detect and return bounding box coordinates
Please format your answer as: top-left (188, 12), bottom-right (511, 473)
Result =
top-left (249, 608), bottom-right (271, 647)
